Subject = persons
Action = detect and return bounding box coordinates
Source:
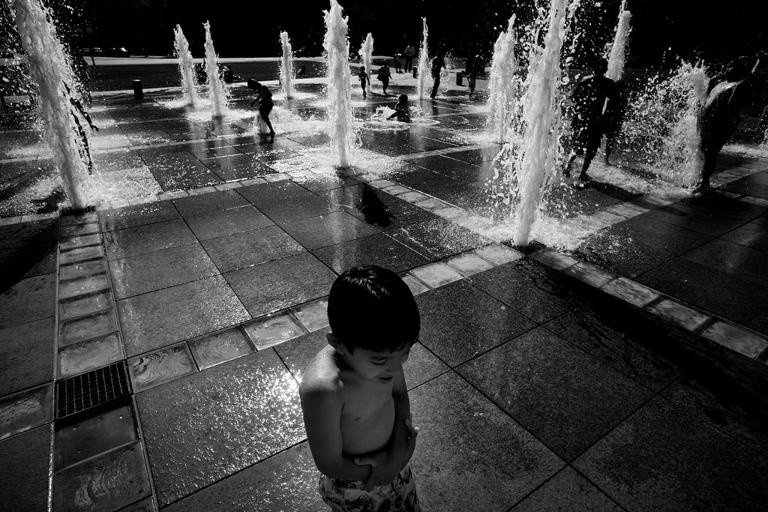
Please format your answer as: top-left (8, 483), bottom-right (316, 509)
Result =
top-left (467, 54), bottom-right (486, 93)
top-left (603, 72), bottom-right (628, 160)
top-left (299, 264), bottom-right (420, 512)
top-left (386, 93), bottom-right (409, 121)
top-left (692, 49), bottom-right (767, 192)
top-left (73, 45), bottom-right (93, 105)
top-left (358, 66), bottom-right (370, 96)
top-left (196, 65), bottom-right (235, 84)
top-left (247, 78), bottom-right (276, 134)
top-left (377, 61), bottom-right (392, 94)
top-left (393, 48), bottom-right (403, 73)
top-left (402, 41), bottom-right (416, 72)
top-left (430, 50), bottom-right (446, 100)
top-left (562, 57), bottom-right (619, 181)
top-left (61, 79), bottom-right (99, 175)
